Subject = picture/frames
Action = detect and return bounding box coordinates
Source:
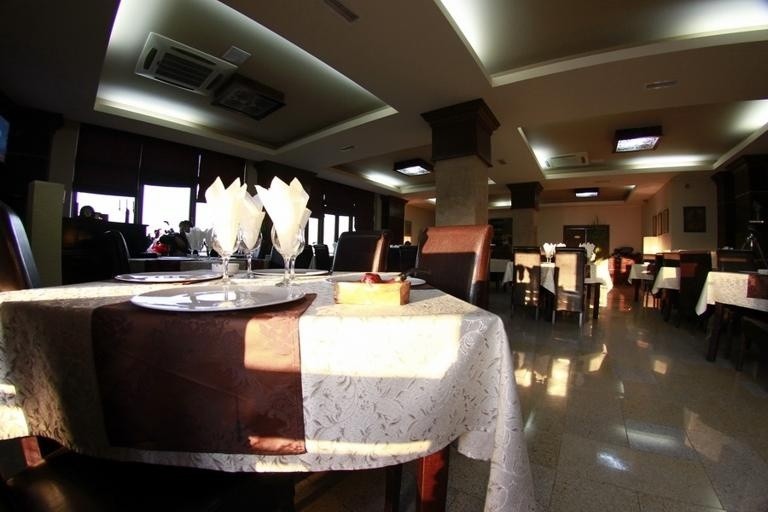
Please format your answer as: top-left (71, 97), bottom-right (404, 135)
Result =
top-left (657, 213), bottom-right (661, 235)
top-left (652, 216), bottom-right (656, 236)
top-left (662, 208), bottom-right (669, 234)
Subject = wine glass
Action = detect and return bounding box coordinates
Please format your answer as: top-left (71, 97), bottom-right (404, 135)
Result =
top-left (544, 250), bottom-right (554, 264)
top-left (271, 223), bottom-right (300, 286)
top-left (237, 223), bottom-right (262, 279)
top-left (211, 223), bottom-right (241, 287)
top-left (284, 224), bottom-right (305, 285)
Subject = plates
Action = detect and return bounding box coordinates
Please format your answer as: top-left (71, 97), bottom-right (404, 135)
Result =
top-left (130, 283), bottom-right (307, 312)
top-left (326, 273), bottom-right (427, 285)
top-left (249, 266), bottom-right (329, 276)
top-left (114, 268), bottom-right (222, 281)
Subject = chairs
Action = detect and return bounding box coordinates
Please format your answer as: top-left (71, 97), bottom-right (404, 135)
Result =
top-left (331, 231), bottom-right (385, 274)
top-left (510, 246), bottom-right (541, 321)
top-left (714, 248), bottom-right (758, 373)
top-left (396, 242), bottom-right (418, 274)
top-left (2, 200), bottom-right (46, 467)
top-left (382, 222), bottom-right (497, 510)
top-left (98, 229), bottom-right (130, 278)
top-left (269, 242), bottom-right (315, 272)
top-left (674, 248), bottom-right (712, 335)
top-left (551, 246), bottom-right (587, 329)
top-left (312, 243), bottom-right (331, 269)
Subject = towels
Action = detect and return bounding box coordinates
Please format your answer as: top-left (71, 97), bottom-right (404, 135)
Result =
top-left (253, 174), bottom-right (311, 251)
top-left (239, 190), bottom-right (265, 249)
top-left (185, 226), bottom-right (213, 251)
top-left (579, 242), bottom-right (595, 259)
top-left (543, 242), bottom-right (566, 258)
top-left (204, 175), bottom-right (247, 251)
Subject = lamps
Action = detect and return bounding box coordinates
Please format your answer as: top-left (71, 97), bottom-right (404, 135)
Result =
top-left (612, 126), bottom-right (663, 153)
top-left (393, 158), bottom-right (433, 177)
top-left (575, 187), bottom-right (599, 198)
top-left (211, 73), bottom-right (286, 121)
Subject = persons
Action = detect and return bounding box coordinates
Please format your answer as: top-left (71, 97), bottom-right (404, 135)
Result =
top-left (156, 220), bottom-right (193, 256)
